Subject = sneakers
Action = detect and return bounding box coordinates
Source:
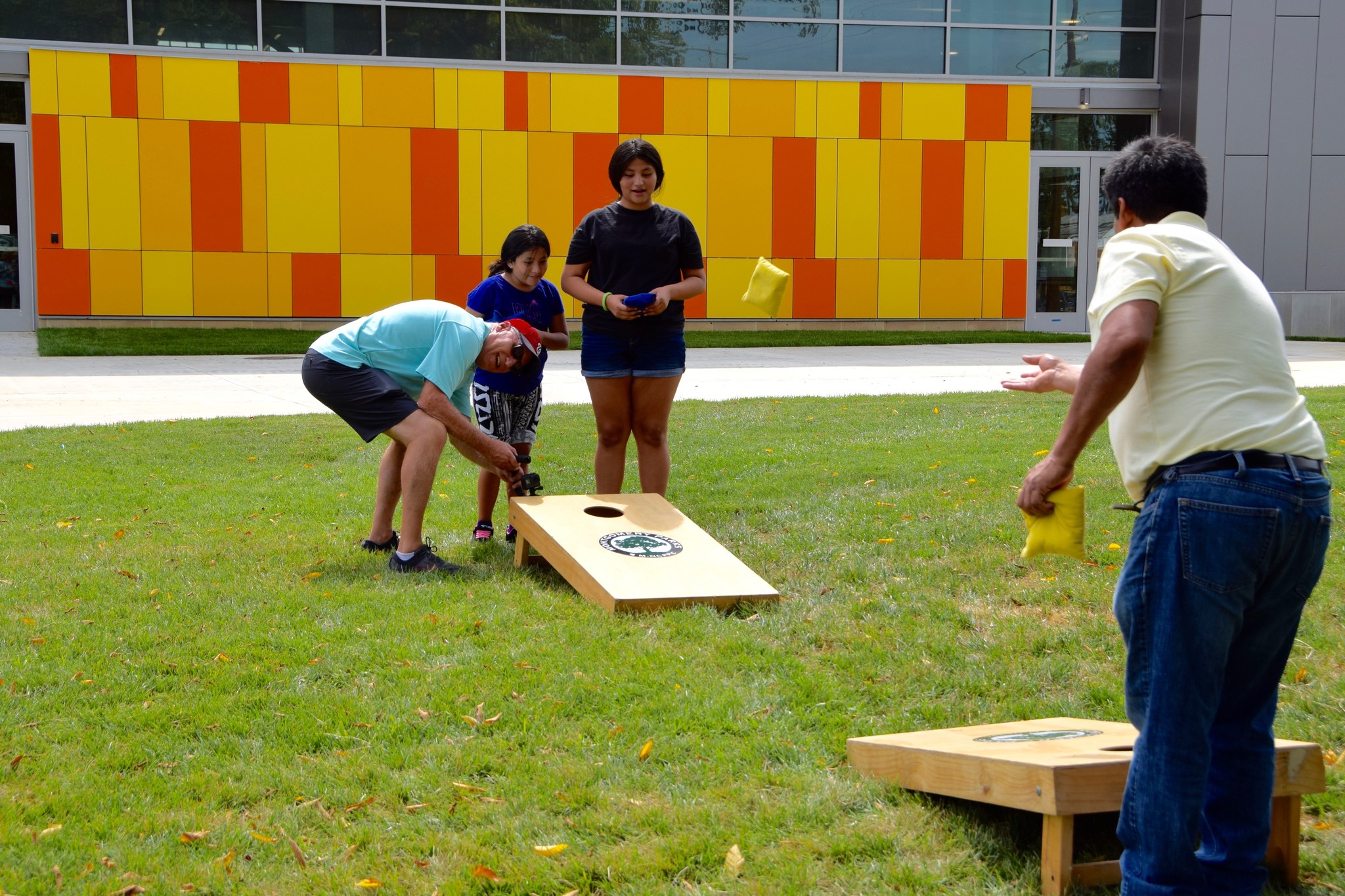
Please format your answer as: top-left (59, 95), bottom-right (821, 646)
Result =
top-left (471, 520), bottom-right (494, 550)
top-left (362, 530), bottom-right (401, 553)
top-left (389, 536), bottom-right (461, 577)
top-left (505, 524), bottom-right (517, 543)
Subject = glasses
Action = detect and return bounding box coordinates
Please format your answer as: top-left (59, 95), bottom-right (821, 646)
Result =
top-left (510, 326), bottom-right (525, 377)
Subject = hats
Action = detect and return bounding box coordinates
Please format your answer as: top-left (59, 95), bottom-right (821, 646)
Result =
top-left (499, 319), bottom-right (542, 368)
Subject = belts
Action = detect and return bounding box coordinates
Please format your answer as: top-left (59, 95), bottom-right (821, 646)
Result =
top-left (1148, 449), bottom-right (1326, 478)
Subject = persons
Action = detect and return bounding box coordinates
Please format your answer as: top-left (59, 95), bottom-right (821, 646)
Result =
top-left (561, 138), bottom-right (705, 499)
top-left (1001, 137), bottom-right (1331, 896)
top-left (302, 299), bottom-right (541, 575)
top-left (464, 223), bottom-right (569, 542)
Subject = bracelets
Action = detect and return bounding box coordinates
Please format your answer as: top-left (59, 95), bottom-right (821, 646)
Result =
top-left (602, 292), bottom-right (613, 311)
